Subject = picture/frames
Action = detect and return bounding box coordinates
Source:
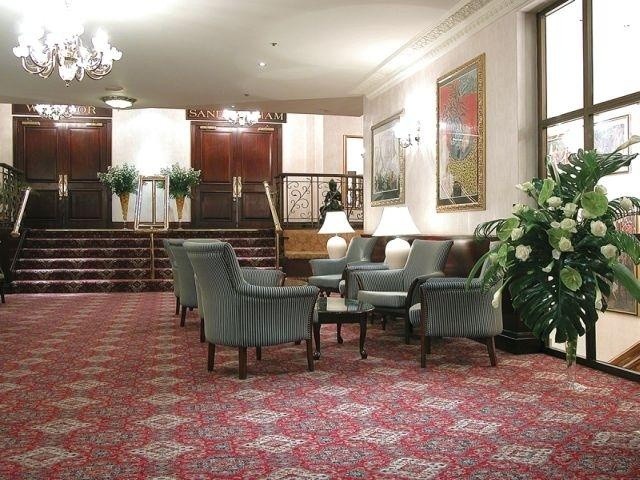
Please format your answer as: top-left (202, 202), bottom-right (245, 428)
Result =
top-left (344, 136), bottom-right (365, 210)
top-left (433, 52), bottom-right (488, 211)
top-left (371, 109), bottom-right (405, 204)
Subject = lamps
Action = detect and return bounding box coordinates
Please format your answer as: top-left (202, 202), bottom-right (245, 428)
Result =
top-left (32, 103), bottom-right (77, 121)
top-left (12, 19), bottom-right (123, 87)
top-left (392, 114), bottom-right (428, 148)
top-left (101, 95), bottom-right (137, 112)
top-left (222, 109), bottom-right (262, 125)
top-left (317, 211), bottom-right (356, 258)
top-left (371, 205), bottom-right (422, 269)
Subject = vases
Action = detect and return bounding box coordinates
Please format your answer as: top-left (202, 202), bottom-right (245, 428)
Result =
top-left (119, 192), bottom-right (130, 230)
top-left (565, 335), bottom-right (577, 391)
top-left (176, 195), bottom-right (185, 231)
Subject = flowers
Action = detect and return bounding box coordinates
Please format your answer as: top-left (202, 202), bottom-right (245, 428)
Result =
top-left (97, 161), bottom-right (140, 196)
top-left (465, 132), bottom-right (640, 363)
top-left (156, 162), bottom-right (203, 200)
